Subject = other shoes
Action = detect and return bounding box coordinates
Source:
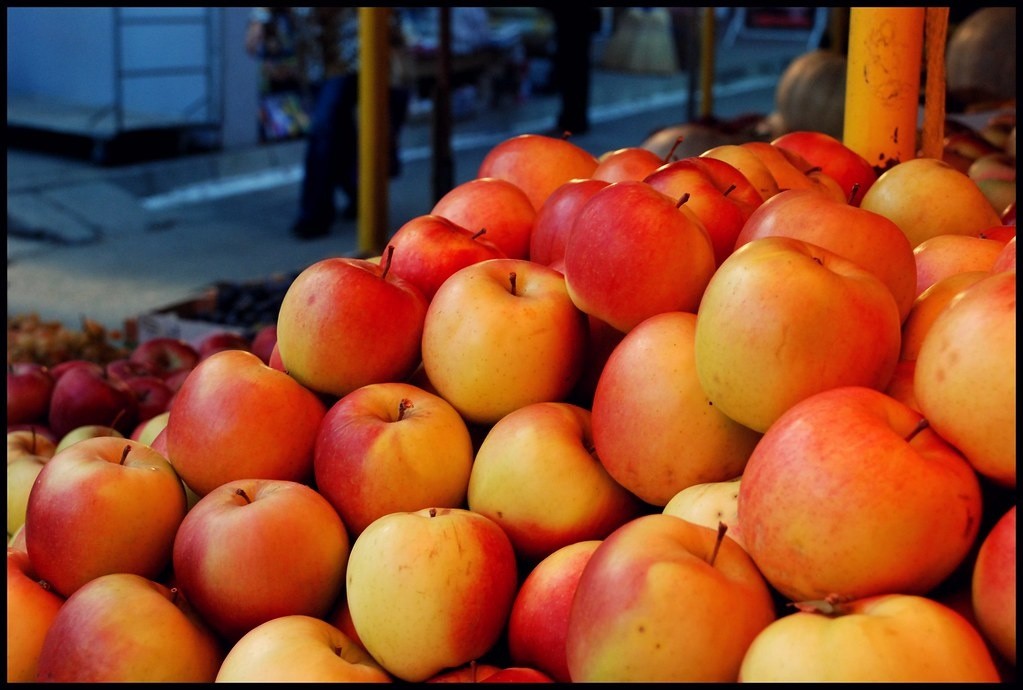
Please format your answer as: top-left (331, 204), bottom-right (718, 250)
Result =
top-left (293, 215), bottom-right (336, 238)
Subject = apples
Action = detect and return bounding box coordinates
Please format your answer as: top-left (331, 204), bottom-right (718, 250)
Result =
top-left (7, 100), bottom-right (1016, 683)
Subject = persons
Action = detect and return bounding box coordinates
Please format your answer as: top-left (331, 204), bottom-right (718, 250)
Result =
top-left (543, 5), bottom-right (605, 139)
top-left (245, 7), bottom-right (409, 242)
